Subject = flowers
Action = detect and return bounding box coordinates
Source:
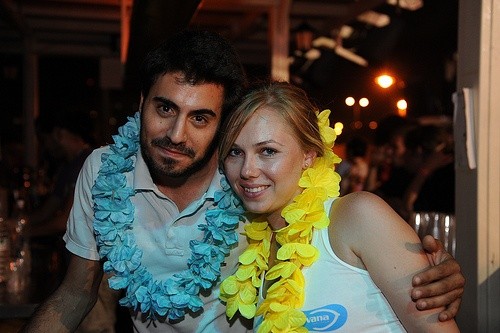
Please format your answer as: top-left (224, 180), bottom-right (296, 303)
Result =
top-left (219, 106), bottom-right (342, 333)
top-left (90, 112), bottom-right (247, 321)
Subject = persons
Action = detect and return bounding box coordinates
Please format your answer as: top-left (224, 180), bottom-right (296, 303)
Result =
top-left (22, 34), bottom-right (466, 333)
top-left (216, 81), bottom-right (461, 333)
top-left (0, 21), bottom-right (457, 259)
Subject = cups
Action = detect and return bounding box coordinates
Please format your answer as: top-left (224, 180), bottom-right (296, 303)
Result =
top-left (411, 213), bottom-right (457, 260)
